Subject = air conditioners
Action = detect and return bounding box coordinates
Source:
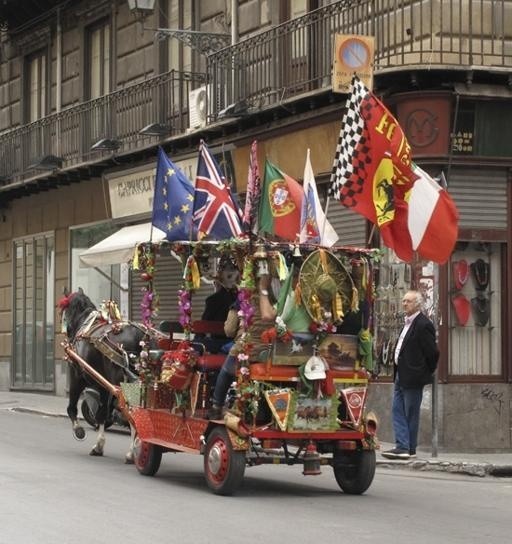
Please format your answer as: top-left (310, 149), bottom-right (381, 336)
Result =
top-left (189, 83), bottom-right (228, 129)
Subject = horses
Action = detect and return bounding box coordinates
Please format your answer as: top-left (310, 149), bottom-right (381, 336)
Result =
top-left (61, 285), bottom-right (161, 465)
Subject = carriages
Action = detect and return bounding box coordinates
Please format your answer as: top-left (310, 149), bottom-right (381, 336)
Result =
top-left (54, 237), bottom-right (384, 494)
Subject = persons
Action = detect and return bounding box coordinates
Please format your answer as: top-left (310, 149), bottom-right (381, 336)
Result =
top-left (381, 290), bottom-right (440, 460)
top-left (203, 297), bottom-right (275, 420)
top-left (258, 269), bottom-right (314, 334)
top-left (191, 264), bottom-right (242, 351)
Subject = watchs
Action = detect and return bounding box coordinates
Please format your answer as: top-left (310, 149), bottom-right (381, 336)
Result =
top-left (258, 288), bottom-right (269, 296)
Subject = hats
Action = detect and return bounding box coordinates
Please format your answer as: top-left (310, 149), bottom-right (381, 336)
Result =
top-left (299, 250), bottom-right (358, 329)
top-left (216, 265), bottom-right (238, 288)
top-left (303, 356), bottom-right (326, 381)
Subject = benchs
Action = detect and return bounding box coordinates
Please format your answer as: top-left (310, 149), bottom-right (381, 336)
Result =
top-left (148, 319), bottom-right (367, 430)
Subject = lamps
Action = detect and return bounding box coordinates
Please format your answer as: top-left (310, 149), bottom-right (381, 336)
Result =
top-left (90, 139), bottom-right (121, 152)
top-left (140, 123), bottom-right (172, 136)
top-left (128, 0), bottom-right (157, 35)
top-left (26, 155), bottom-right (65, 170)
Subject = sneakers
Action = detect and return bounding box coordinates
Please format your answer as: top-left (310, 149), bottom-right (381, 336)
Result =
top-left (203, 405), bottom-right (223, 420)
top-left (382, 446), bottom-right (416, 459)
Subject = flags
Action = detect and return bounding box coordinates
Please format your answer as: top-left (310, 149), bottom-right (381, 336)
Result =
top-left (409, 163), bottom-right (460, 265)
top-left (259, 157), bottom-right (304, 240)
top-left (152, 145), bottom-right (195, 242)
top-left (327, 74), bottom-right (415, 265)
top-left (244, 138), bottom-right (261, 235)
top-left (191, 142), bottom-right (245, 240)
top-left (299, 149), bottom-right (340, 248)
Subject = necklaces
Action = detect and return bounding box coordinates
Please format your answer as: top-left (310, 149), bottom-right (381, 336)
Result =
top-left (457, 263), bottom-right (469, 284)
top-left (474, 263), bottom-right (488, 285)
top-left (476, 302), bottom-right (488, 314)
